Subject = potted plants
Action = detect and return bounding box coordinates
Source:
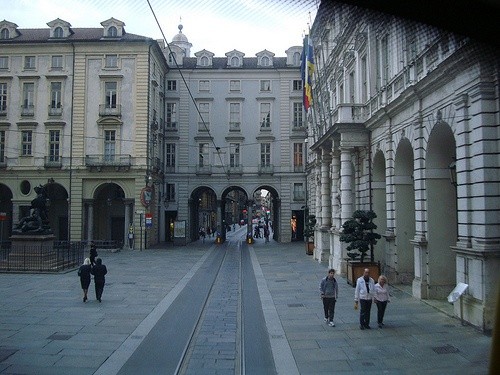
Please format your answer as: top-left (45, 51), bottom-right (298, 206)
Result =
top-left (303, 214), bottom-right (317, 256)
top-left (339, 208), bottom-right (382, 287)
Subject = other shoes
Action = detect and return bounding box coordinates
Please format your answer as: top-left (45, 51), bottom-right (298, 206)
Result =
top-left (360, 326), bottom-right (364, 330)
top-left (377, 322), bottom-right (384, 328)
top-left (85, 296), bottom-right (87, 300)
top-left (83, 297), bottom-right (86, 302)
top-left (364, 325), bottom-right (370, 329)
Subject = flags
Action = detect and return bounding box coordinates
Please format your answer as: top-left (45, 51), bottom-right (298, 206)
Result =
top-left (300, 32), bottom-right (316, 113)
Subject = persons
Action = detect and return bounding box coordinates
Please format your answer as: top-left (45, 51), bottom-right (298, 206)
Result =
top-left (320, 268), bottom-right (339, 327)
top-left (354, 267), bottom-right (375, 329)
top-left (32, 186), bottom-right (49, 230)
top-left (77, 257), bottom-right (93, 303)
top-left (89, 245), bottom-right (98, 266)
top-left (199, 226), bottom-right (216, 238)
top-left (12, 209), bottom-right (45, 233)
top-left (253, 225), bottom-right (262, 238)
top-left (373, 275), bottom-right (390, 328)
top-left (91, 257), bottom-right (107, 303)
top-left (90, 241), bottom-right (94, 246)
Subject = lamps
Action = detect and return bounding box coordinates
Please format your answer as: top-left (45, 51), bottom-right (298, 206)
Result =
top-left (449, 162), bottom-right (457, 187)
top-left (164, 199), bottom-right (170, 209)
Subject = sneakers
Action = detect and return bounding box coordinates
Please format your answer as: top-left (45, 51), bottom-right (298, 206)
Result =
top-left (329, 321), bottom-right (335, 327)
top-left (324, 317), bottom-right (328, 323)
